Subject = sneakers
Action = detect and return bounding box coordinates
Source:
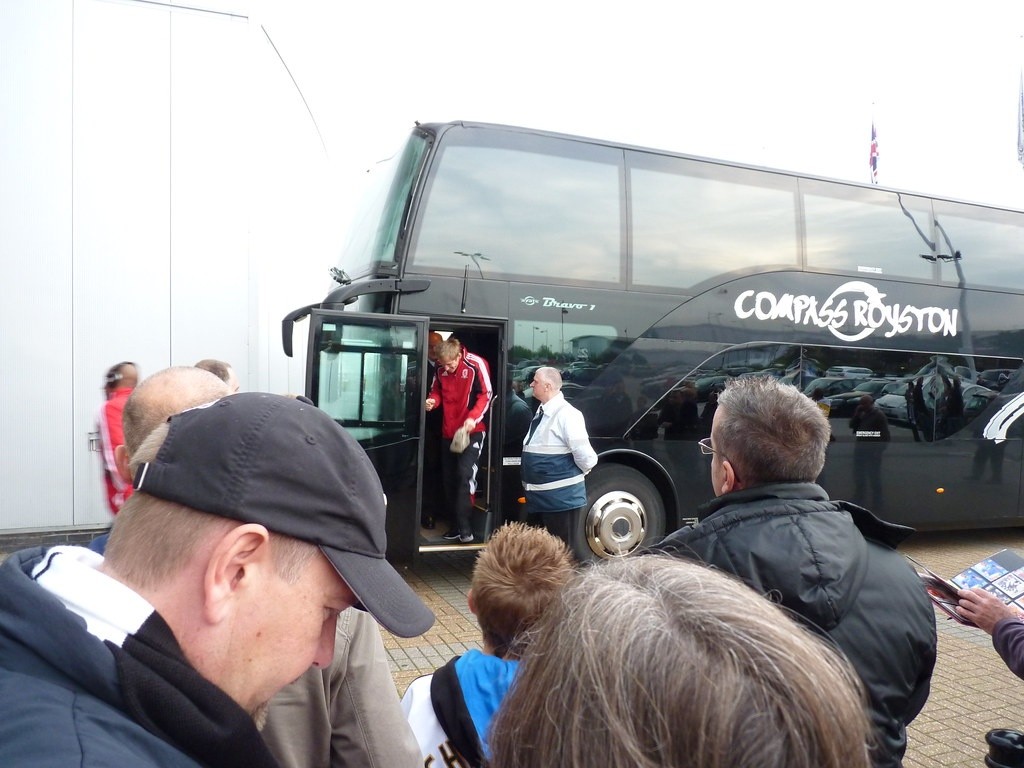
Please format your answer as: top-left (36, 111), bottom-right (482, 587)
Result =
top-left (460, 531), bottom-right (475, 543)
top-left (442, 528), bottom-right (459, 540)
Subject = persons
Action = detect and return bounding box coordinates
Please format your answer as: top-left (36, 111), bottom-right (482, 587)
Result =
top-left (955, 587), bottom-right (1024, 680)
top-left (638, 376), bottom-right (936, 768)
top-left (503, 358), bottom-right (1011, 510)
top-left (518, 366), bottom-right (599, 551)
top-left (115, 367), bottom-right (426, 768)
top-left (400, 522), bottom-right (575, 768)
top-left (482, 557), bottom-right (869, 768)
top-left (407, 331), bottom-right (494, 542)
top-left (97, 359), bottom-right (239, 520)
top-left (0, 391), bottom-right (435, 768)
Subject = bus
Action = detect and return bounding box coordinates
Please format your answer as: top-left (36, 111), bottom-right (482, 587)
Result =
top-left (281, 120), bottom-right (1024, 572)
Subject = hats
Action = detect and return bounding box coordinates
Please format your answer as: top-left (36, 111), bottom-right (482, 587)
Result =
top-left (129, 391), bottom-right (435, 637)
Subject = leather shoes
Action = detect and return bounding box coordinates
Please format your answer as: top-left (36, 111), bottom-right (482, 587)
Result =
top-left (421, 510), bottom-right (435, 529)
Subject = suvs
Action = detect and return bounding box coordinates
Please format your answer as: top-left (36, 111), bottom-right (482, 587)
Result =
top-left (826, 366), bottom-right (876, 379)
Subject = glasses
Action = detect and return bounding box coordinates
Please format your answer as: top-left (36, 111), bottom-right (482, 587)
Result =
top-left (699, 438), bottom-right (740, 485)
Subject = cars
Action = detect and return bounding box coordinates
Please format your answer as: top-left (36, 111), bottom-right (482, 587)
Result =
top-left (817, 381), bottom-right (894, 416)
top-left (977, 368), bottom-right (1014, 389)
top-left (803, 377), bottom-right (870, 400)
top-left (872, 377), bottom-right (995, 426)
